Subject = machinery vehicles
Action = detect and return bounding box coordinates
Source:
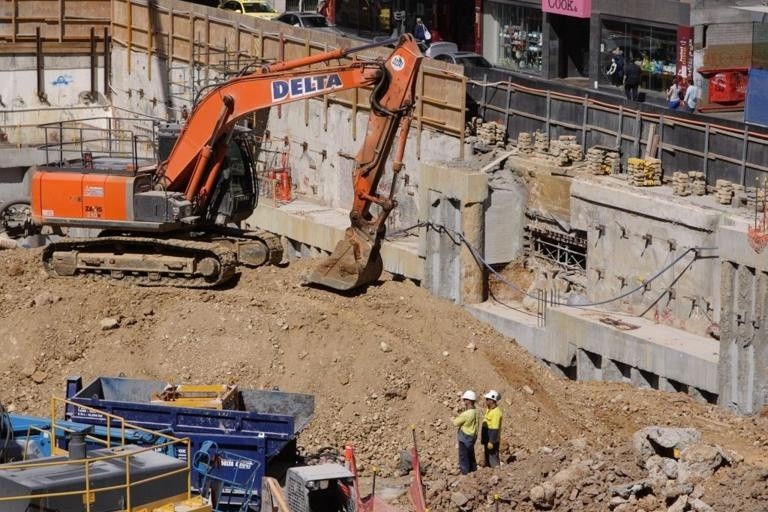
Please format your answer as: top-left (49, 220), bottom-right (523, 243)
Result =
top-left (30, 32), bottom-right (424, 290)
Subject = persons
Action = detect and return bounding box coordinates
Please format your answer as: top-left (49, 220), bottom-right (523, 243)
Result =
top-left (316, 1), bottom-right (329, 17)
top-left (336, 1), bottom-right (386, 38)
top-left (607, 47), bottom-right (624, 83)
top-left (623, 57), bottom-right (643, 103)
top-left (683, 78), bottom-right (698, 114)
top-left (449, 390), bottom-right (480, 476)
top-left (666, 78), bottom-right (682, 112)
top-left (481, 389), bottom-right (503, 468)
top-left (403, 12), bottom-right (442, 46)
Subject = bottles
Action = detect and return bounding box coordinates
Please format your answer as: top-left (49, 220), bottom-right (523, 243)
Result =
top-left (519, 56), bottom-right (543, 73)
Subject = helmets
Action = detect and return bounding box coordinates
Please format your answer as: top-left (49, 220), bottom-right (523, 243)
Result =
top-left (462, 389), bottom-right (476, 401)
top-left (485, 391), bottom-right (500, 401)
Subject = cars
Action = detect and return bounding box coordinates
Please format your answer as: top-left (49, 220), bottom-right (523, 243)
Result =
top-left (216, 0), bottom-right (348, 38)
top-left (373, 37), bottom-right (492, 68)
